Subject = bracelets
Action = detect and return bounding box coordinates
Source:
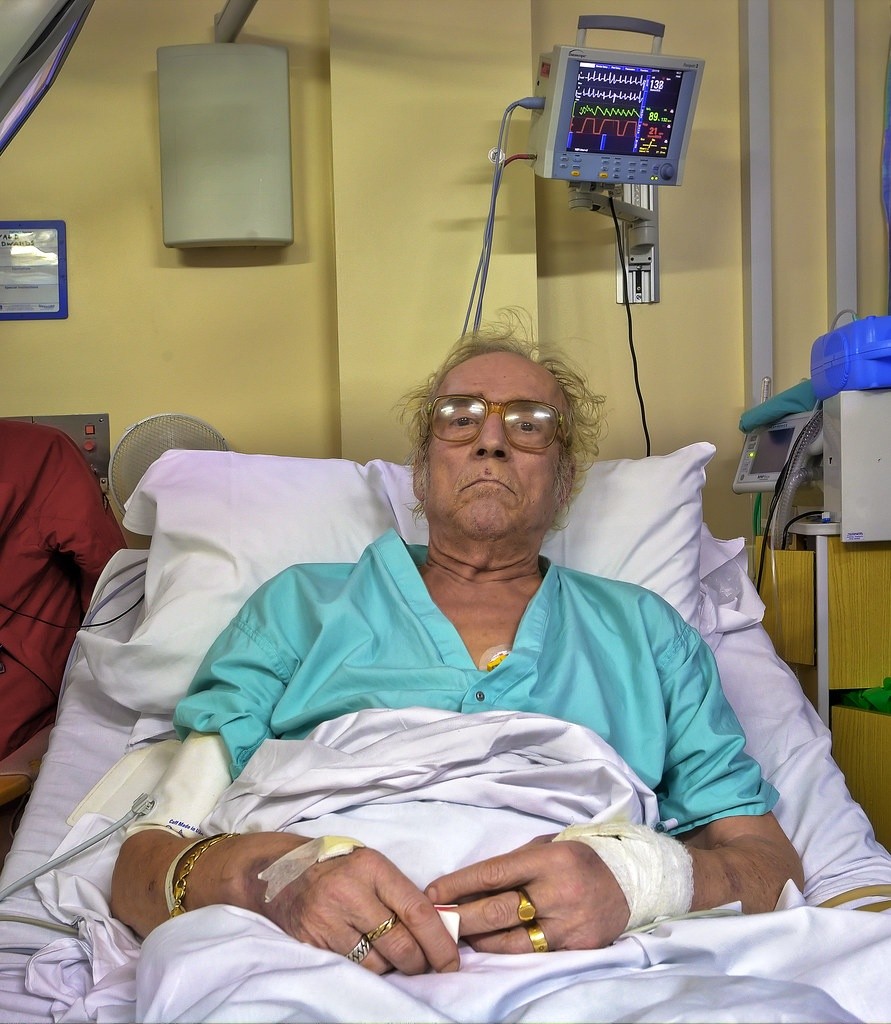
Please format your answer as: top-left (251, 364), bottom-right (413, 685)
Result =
top-left (164, 832), bottom-right (241, 918)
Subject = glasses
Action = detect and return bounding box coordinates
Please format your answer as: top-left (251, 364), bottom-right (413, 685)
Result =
top-left (426, 395), bottom-right (570, 451)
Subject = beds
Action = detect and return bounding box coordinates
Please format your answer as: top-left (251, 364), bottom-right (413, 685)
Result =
top-left (0, 524), bottom-right (891, 1024)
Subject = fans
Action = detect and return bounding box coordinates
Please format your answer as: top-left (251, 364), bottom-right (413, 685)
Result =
top-left (108, 412), bottom-right (229, 521)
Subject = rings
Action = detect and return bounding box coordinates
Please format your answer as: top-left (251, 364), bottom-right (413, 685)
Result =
top-left (524, 921), bottom-right (548, 952)
top-left (514, 885), bottom-right (536, 921)
top-left (366, 911), bottom-right (400, 941)
top-left (344, 933), bottom-right (373, 963)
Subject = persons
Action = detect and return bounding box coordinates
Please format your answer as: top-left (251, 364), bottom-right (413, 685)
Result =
top-left (109, 327), bottom-right (807, 977)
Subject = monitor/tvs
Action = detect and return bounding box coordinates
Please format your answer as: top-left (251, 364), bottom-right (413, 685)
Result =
top-left (733, 410), bottom-right (816, 494)
top-left (524, 43), bottom-right (706, 186)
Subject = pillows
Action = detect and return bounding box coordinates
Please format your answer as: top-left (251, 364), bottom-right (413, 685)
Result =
top-left (77, 442), bottom-right (717, 714)
